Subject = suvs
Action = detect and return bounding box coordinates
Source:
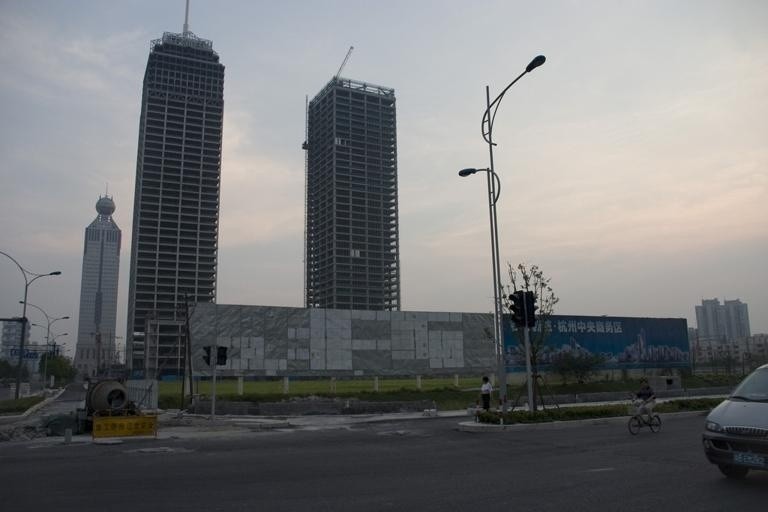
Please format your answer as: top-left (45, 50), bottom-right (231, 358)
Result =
top-left (702, 363), bottom-right (768, 479)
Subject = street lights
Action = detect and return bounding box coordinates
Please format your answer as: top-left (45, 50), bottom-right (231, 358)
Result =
top-left (0, 251), bottom-right (73, 400)
top-left (457, 53), bottom-right (547, 435)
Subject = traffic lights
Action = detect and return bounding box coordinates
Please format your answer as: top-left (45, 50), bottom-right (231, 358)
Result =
top-left (202, 345), bottom-right (212, 366)
top-left (526, 291), bottom-right (539, 327)
top-left (509, 290), bottom-right (526, 328)
top-left (216, 346), bottom-right (228, 365)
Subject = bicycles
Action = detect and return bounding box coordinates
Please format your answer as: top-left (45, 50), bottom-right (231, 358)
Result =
top-left (629, 402), bottom-right (663, 435)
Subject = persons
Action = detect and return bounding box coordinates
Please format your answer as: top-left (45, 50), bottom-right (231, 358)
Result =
top-left (631, 376), bottom-right (656, 421)
top-left (480, 375), bottom-right (493, 411)
